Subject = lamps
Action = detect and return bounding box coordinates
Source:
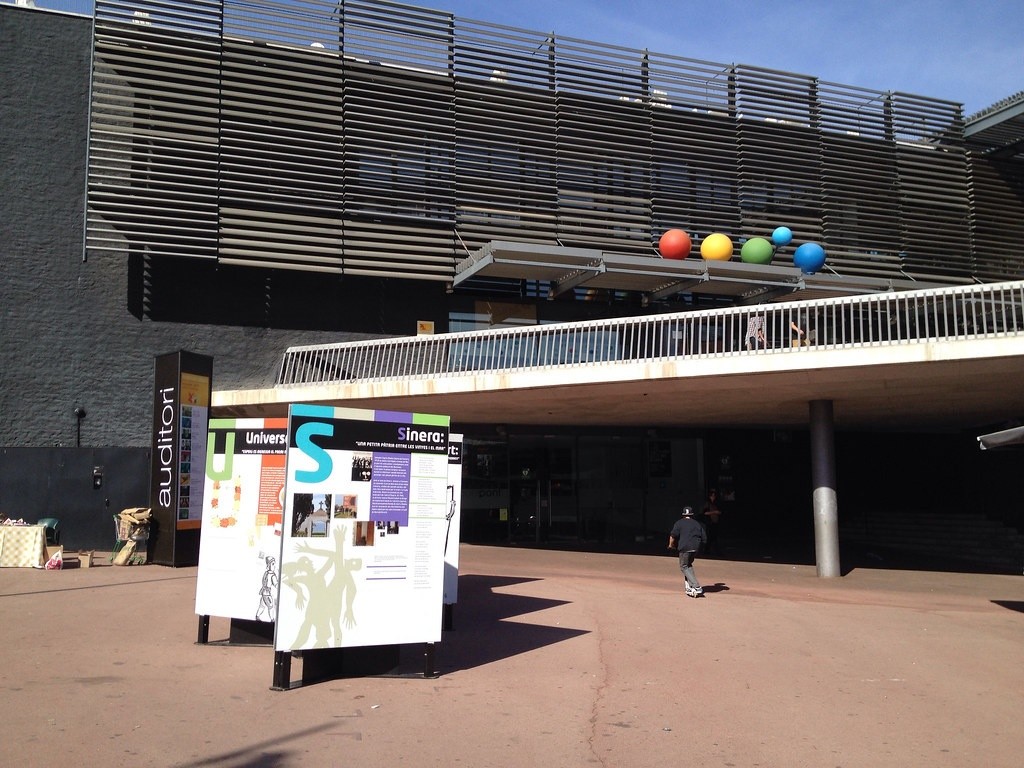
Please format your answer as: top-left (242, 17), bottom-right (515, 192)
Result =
top-left (74, 407), bottom-right (86, 446)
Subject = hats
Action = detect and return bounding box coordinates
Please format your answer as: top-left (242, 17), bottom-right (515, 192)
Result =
top-left (682, 507), bottom-right (693, 516)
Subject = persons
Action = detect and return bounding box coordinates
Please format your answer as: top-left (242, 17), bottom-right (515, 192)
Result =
top-left (791, 298), bottom-right (811, 347)
top-left (745, 312), bottom-right (768, 350)
top-left (668, 507), bottom-right (707, 598)
top-left (704, 491), bottom-right (722, 555)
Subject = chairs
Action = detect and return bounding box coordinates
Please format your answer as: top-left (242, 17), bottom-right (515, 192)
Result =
top-left (37, 518), bottom-right (61, 545)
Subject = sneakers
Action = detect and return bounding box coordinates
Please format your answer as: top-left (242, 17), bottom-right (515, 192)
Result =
top-left (686, 587), bottom-right (703, 594)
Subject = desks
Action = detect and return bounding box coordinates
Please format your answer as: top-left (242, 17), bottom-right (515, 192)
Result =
top-left (0, 523), bottom-right (49, 569)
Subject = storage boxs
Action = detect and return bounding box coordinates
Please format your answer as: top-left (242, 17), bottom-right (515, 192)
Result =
top-left (111, 508), bottom-right (153, 566)
top-left (44, 544), bottom-right (63, 564)
top-left (78, 548), bottom-right (95, 568)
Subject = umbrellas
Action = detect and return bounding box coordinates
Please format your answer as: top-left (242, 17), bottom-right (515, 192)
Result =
top-left (977, 426), bottom-right (1024, 450)
